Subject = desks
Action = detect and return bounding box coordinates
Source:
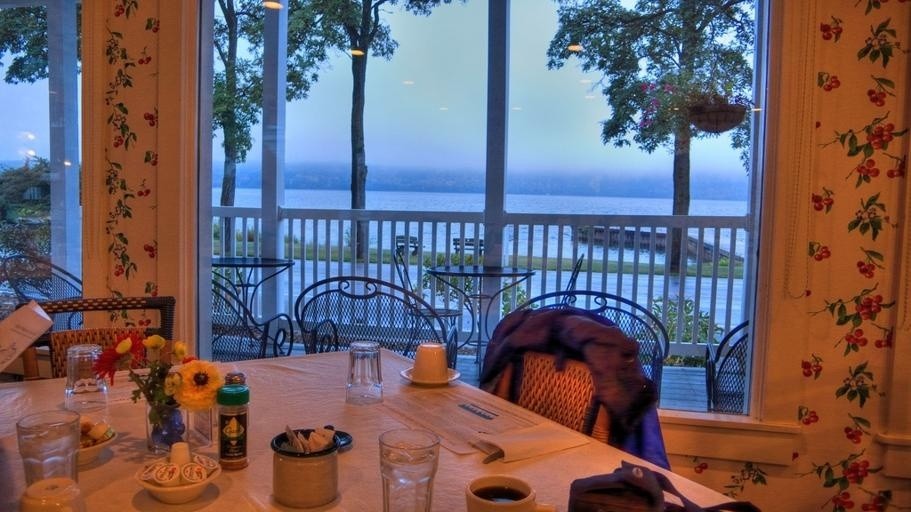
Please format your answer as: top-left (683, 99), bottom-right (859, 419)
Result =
top-left (426, 265), bottom-right (536, 381)
top-left (212, 257), bottom-right (294, 347)
top-left (0, 349), bottom-right (736, 512)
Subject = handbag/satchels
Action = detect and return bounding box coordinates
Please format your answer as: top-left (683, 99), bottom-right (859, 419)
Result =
top-left (568, 461), bottom-right (664, 512)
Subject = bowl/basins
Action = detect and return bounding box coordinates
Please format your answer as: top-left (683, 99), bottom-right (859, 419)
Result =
top-left (78, 429), bottom-right (119, 467)
top-left (135, 452), bottom-right (220, 502)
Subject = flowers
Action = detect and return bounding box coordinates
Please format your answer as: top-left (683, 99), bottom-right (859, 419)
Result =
top-left (91, 332), bottom-right (224, 410)
top-left (629, 73), bottom-right (734, 151)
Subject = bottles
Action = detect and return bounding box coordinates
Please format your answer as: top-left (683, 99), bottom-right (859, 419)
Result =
top-left (225, 369), bottom-right (248, 387)
top-left (214, 384), bottom-right (251, 470)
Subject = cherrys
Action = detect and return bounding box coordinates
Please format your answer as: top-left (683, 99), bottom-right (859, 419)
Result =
top-left (105, 3), bottom-right (159, 326)
top-left (798, 19), bottom-right (906, 512)
top-left (694, 457), bottom-right (709, 474)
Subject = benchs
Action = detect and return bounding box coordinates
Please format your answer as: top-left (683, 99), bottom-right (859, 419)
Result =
top-left (395, 236), bottom-right (425, 256)
top-left (453, 237), bottom-right (483, 256)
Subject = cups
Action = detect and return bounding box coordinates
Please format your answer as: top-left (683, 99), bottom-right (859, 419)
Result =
top-left (21, 475), bottom-right (88, 511)
top-left (345, 340), bottom-right (384, 404)
top-left (466, 474), bottom-right (556, 511)
top-left (16, 405), bottom-right (83, 481)
top-left (412, 343), bottom-right (450, 381)
top-left (65, 343), bottom-right (110, 411)
top-left (378, 427), bottom-right (441, 509)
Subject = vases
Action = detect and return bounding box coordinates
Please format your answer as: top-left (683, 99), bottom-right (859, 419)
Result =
top-left (145, 401), bottom-right (185, 453)
top-left (688, 104), bottom-right (746, 133)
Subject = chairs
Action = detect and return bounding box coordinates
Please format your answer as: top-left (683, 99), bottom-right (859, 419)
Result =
top-left (294, 275), bottom-right (457, 371)
top-left (212, 271), bottom-right (293, 362)
top-left (704, 321), bottom-right (750, 415)
top-left (393, 248), bottom-right (462, 357)
top-left (560, 254), bottom-right (584, 304)
top-left (515, 290), bottom-right (670, 408)
top-left (478, 308), bottom-right (670, 471)
top-left (15, 296), bottom-right (176, 381)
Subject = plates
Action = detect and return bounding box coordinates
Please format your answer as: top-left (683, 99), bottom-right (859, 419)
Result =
top-left (401, 366), bottom-right (460, 387)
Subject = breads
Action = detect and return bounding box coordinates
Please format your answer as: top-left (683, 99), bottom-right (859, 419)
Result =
top-left (80, 416), bottom-right (114, 447)
